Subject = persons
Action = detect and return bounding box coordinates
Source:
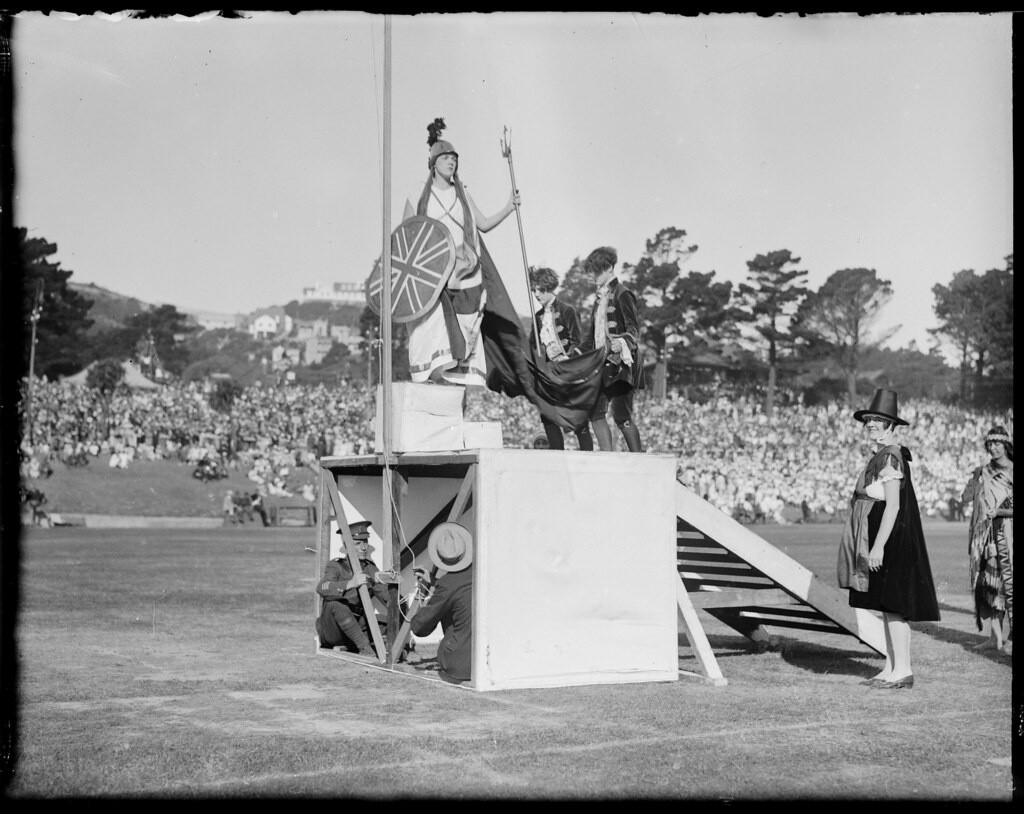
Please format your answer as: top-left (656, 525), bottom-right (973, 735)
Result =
top-left (403, 118), bottom-right (522, 388)
top-left (18, 376), bottom-right (1015, 523)
top-left (566, 246), bottom-right (646, 453)
top-left (528, 268), bottom-right (593, 451)
top-left (838, 390), bottom-right (941, 689)
top-left (410, 522), bottom-right (473, 680)
top-left (962, 426), bottom-right (1013, 655)
top-left (316, 523), bottom-right (422, 664)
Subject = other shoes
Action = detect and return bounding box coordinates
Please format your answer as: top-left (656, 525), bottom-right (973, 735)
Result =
top-left (871, 673), bottom-right (914, 689)
top-left (973, 641), bottom-right (997, 649)
top-left (859, 678), bottom-right (880, 685)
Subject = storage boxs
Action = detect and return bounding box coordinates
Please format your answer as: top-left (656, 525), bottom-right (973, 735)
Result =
top-left (374, 382), bottom-right (504, 454)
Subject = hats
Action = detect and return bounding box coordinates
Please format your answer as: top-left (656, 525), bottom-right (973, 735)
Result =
top-left (428, 521), bottom-right (474, 571)
top-left (336, 520), bottom-right (371, 540)
top-left (854, 389), bottom-right (910, 425)
